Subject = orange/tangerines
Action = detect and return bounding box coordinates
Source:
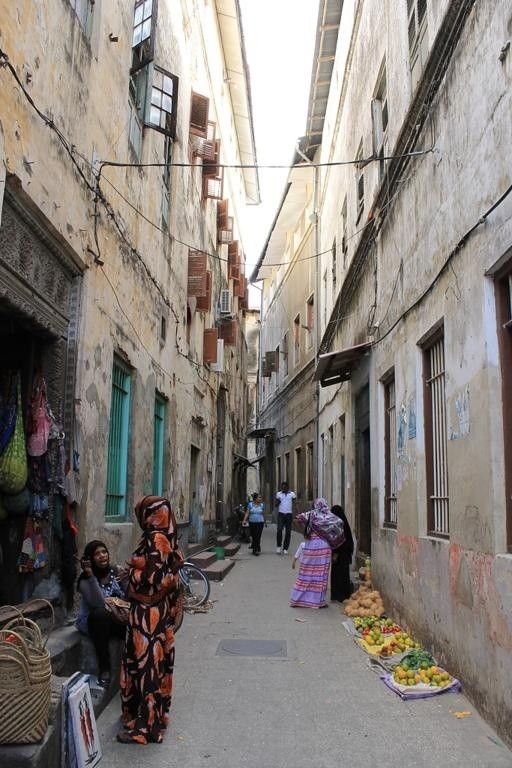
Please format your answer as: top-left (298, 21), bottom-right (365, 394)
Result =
top-left (353, 614), bottom-right (451, 689)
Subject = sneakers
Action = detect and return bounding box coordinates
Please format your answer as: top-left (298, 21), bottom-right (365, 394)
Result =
top-left (253, 549), bottom-right (259, 556)
top-left (248, 544), bottom-right (254, 549)
top-left (283, 549), bottom-right (288, 555)
top-left (276, 546), bottom-right (281, 554)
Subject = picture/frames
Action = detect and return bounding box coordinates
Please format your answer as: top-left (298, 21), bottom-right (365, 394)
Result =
top-left (60, 671), bottom-right (103, 768)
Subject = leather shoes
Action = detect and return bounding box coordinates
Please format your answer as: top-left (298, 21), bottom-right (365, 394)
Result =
top-left (96, 672), bottom-right (112, 689)
top-left (116, 732), bottom-right (144, 744)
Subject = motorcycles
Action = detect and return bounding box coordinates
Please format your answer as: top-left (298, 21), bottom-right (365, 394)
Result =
top-left (233, 500), bottom-right (253, 544)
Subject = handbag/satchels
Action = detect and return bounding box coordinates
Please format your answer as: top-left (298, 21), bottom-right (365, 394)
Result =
top-left (1, 368), bottom-right (80, 546)
top-left (303, 512), bottom-right (312, 540)
top-left (128, 583), bottom-right (170, 605)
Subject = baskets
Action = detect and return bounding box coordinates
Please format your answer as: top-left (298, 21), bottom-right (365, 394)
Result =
top-left (0, 597), bottom-right (55, 745)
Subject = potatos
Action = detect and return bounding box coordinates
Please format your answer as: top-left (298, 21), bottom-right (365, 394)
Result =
top-left (343, 585), bottom-right (385, 618)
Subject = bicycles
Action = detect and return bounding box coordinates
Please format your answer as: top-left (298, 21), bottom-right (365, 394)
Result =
top-left (172, 535), bottom-right (210, 610)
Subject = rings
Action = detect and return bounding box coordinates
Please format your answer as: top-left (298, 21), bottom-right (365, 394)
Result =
top-left (113, 599), bottom-right (120, 603)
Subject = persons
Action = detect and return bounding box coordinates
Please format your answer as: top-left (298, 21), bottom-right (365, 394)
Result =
top-left (76, 539), bottom-right (128, 689)
top-left (330, 505), bottom-right (355, 603)
top-left (289, 496), bottom-right (348, 610)
top-left (291, 541), bottom-right (305, 568)
top-left (274, 481), bottom-right (297, 556)
top-left (242, 491), bottom-right (268, 557)
top-left (117, 493), bottom-right (185, 744)
